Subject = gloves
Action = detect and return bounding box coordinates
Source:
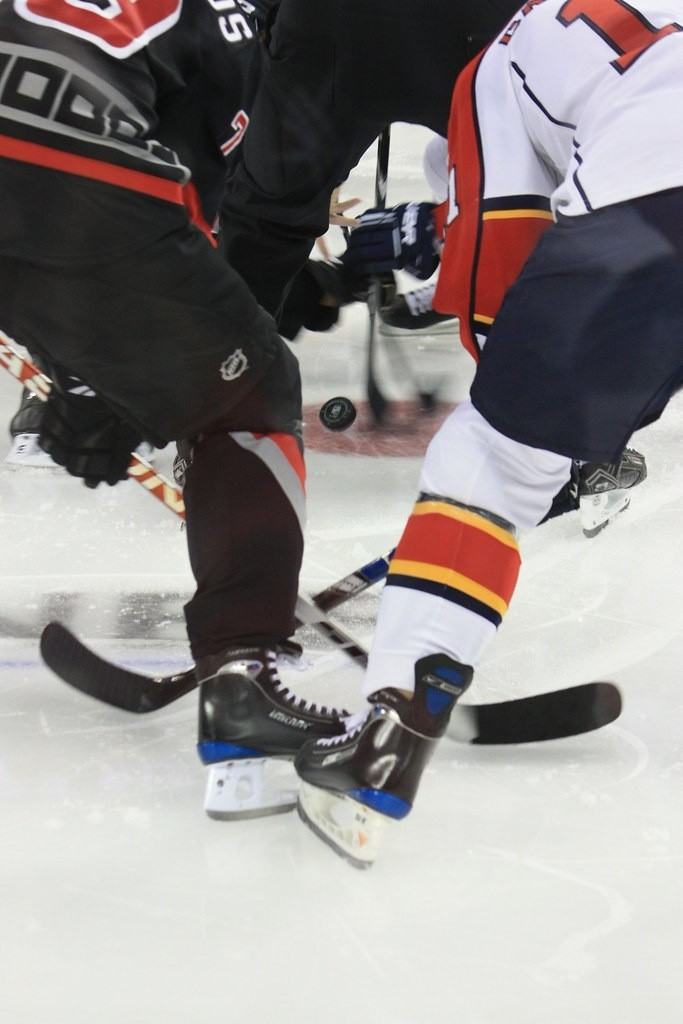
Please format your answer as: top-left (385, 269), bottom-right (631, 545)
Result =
top-left (350, 199), bottom-right (440, 281)
top-left (35, 363), bottom-right (143, 490)
top-left (303, 233), bottom-right (398, 310)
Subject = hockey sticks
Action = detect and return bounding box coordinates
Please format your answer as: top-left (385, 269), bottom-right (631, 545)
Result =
top-left (364, 121), bottom-right (391, 418)
top-left (3, 337), bottom-right (624, 749)
top-left (36, 485), bottom-right (583, 716)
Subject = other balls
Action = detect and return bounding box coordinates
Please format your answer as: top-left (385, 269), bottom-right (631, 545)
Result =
top-left (317, 395), bottom-right (357, 434)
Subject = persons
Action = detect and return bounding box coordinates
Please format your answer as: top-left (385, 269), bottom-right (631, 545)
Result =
top-left (1, 2), bottom-right (365, 820)
top-left (4, 123), bottom-right (448, 476)
top-left (36, 0), bottom-right (683, 864)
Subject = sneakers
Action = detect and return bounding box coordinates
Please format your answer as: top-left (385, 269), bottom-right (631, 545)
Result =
top-left (192, 639), bottom-right (359, 821)
top-left (5, 353), bottom-right (98, 468)
top-left (377, 281), bottom-right (461, 337)
top-left (570, 445), bottom-right (648, 540)
top-left (293, 652), bottom-right (473, 870)
